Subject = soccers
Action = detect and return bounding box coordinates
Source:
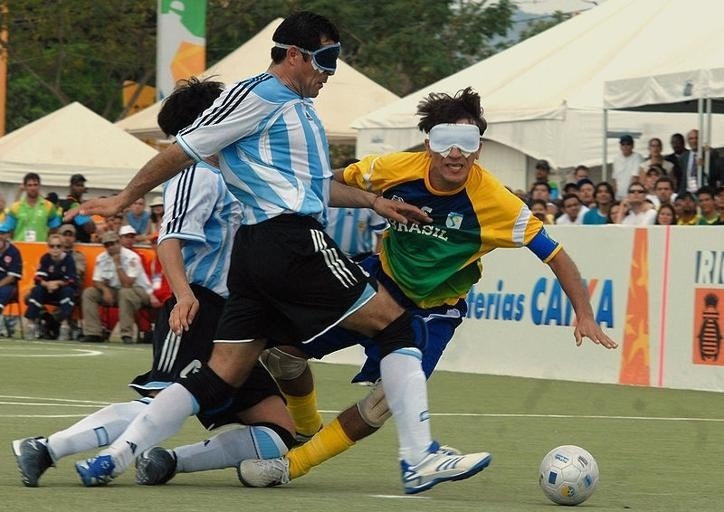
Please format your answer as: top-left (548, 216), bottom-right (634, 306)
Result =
top-left (537, 444), bottom-right (599, 505)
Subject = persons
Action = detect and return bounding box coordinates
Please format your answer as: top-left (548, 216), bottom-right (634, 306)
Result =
top-left (237, 83), bottom-right (615, 488)
top-left (0, 172), bottom-right (173, 344)
top-left (504, 129), bottom-right (724, 225)
top-left (63, 10), bottom-right (489, 494)
top-left (10, 73), bottom-right (297, 484)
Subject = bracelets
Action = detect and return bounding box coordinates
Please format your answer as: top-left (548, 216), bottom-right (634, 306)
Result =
top-left (372, 195), bottom-right (381, 210)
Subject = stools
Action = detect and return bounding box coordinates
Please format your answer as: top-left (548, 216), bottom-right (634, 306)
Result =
top-left (0, 274), bottom-right (154, 344)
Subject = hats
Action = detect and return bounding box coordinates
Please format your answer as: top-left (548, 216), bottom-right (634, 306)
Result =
top-left (102, 231), bottom-right (120, 243)
top-left (57, 224), bottom-right (76, 234)
top-left (71, 174), bottom-right (86, 185)
top-left (149, 196), bottom-right (164, 207)
top-left (119, 225), bottom-right (137, 236)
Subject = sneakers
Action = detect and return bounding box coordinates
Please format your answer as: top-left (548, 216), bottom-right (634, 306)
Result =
top-left (75, 455), bottom-right (119, 487)
top-left (11, 435), bottom-right (56, 488)
top-left (237, 455), bottom-right (292, 488)
top-left (134, 447), bottom-right (177, 485)
top-left (399, 441), bottom-right (491, 494)
